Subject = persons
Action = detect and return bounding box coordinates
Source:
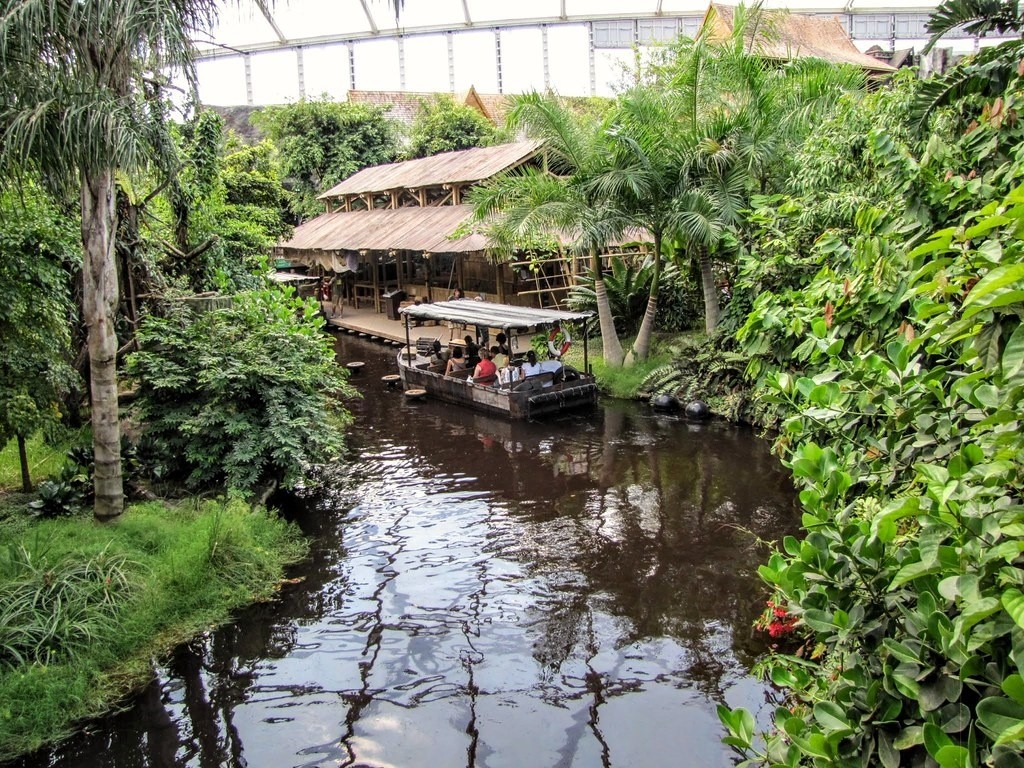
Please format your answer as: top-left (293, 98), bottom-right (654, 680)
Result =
top-left (491, 345), bottom-right (510, 372)
top-left (474, 295), bottom-right (490, 350)
top-left (467, 348), bottom-right (503, 387)
top-left (448, 288), bottom-right (465, 341)
top-left (493, 356), bottom-right (519, 387)
top-left (464, 335), bottom-right (482, 368)
top-left (430, 340), bottom-right (448, 367)
top-left (444, 346), bottom-right (467, 379)
top-left (520, 350), bottom-right (540, 380)
top-left (328, 273), bottom-right (345, 319)
top-left (540, 350), bottom-right (563, 387)
top-left (495, 333), bottom-right (514, 360)
top-left (281, 269), bottom-right (300, 301)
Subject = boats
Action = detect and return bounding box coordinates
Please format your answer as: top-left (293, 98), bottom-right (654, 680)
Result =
top-left (397, 297), bottom-right (596, 421)
top-left (268, 271), bottom-right (329, 330)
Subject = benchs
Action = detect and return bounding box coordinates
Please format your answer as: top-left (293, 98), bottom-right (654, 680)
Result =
top-left (428, 356), bottom-right (555, 390)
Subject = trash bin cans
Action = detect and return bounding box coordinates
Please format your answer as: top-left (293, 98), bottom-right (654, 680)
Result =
top-left (382, 289), bottom-right (403, 321)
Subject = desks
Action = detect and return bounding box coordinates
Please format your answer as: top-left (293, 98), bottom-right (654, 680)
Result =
top-left (354, 282), bottom-right (397, 313)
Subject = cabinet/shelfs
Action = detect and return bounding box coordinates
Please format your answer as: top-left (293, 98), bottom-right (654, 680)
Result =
top-left (385, 292), bottom-right (406, 321)
